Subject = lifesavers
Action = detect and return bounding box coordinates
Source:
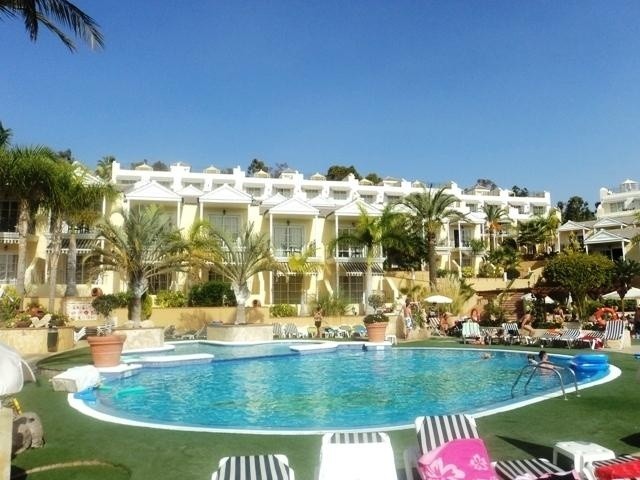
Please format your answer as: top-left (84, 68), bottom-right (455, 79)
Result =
top-left (570, 355), bottom-right (609, 371)
top-left (596, 308), bottom-right (618, 327)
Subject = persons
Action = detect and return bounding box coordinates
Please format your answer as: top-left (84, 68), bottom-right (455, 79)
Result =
top-left (545, 302), bottom-right (640, 335)
top-left (402, 298), bottom-right (412, 330)
top-left (313, 306), bottom-right (323, 339)
top-left (428, 302), bottom-right (438, 320)
top-left (527, 353), bottom-right (539, 370)
top-left (537, 350), bottom-right (564, 380)
top-left (438, 313), bottom-right (449, 335)
top-left (519, 310), bottom-right (537, 337)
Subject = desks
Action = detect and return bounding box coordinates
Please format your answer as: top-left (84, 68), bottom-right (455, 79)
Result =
top-left (553, 439), bottom-right (616, 471)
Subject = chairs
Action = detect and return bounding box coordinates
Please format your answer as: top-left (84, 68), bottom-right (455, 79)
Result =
top-left (583, 454), bottom-right (639, 480)
top-left (401, 414), bottom-right (499, 479)
top-left (165, 321), bottom-right (209, 342)
top-left (272, 321), bottom-right (367, 342)
top-left (212, 453), bottom-right (294, 479)
top-left (491, 457), bottom-right (578, 479)
top-left (314, 430), bottom-right (400, 479)
top-left (424, 319), bottom-right (631, 349)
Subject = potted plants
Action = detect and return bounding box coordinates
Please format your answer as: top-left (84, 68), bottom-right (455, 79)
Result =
top-left (87, 292), bottom-right (127, 367)
top-left (363, 313), bottom-right (389, 342)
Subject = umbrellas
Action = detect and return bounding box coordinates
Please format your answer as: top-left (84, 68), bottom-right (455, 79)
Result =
top-left (566, 291), bottom-right (574, 308)
top-left (600, 286), bottom-right (640, 300)
top-left (520, 292), bottom-right (555, 304)
top-left (424, 295), bottom-right (453, 304)
